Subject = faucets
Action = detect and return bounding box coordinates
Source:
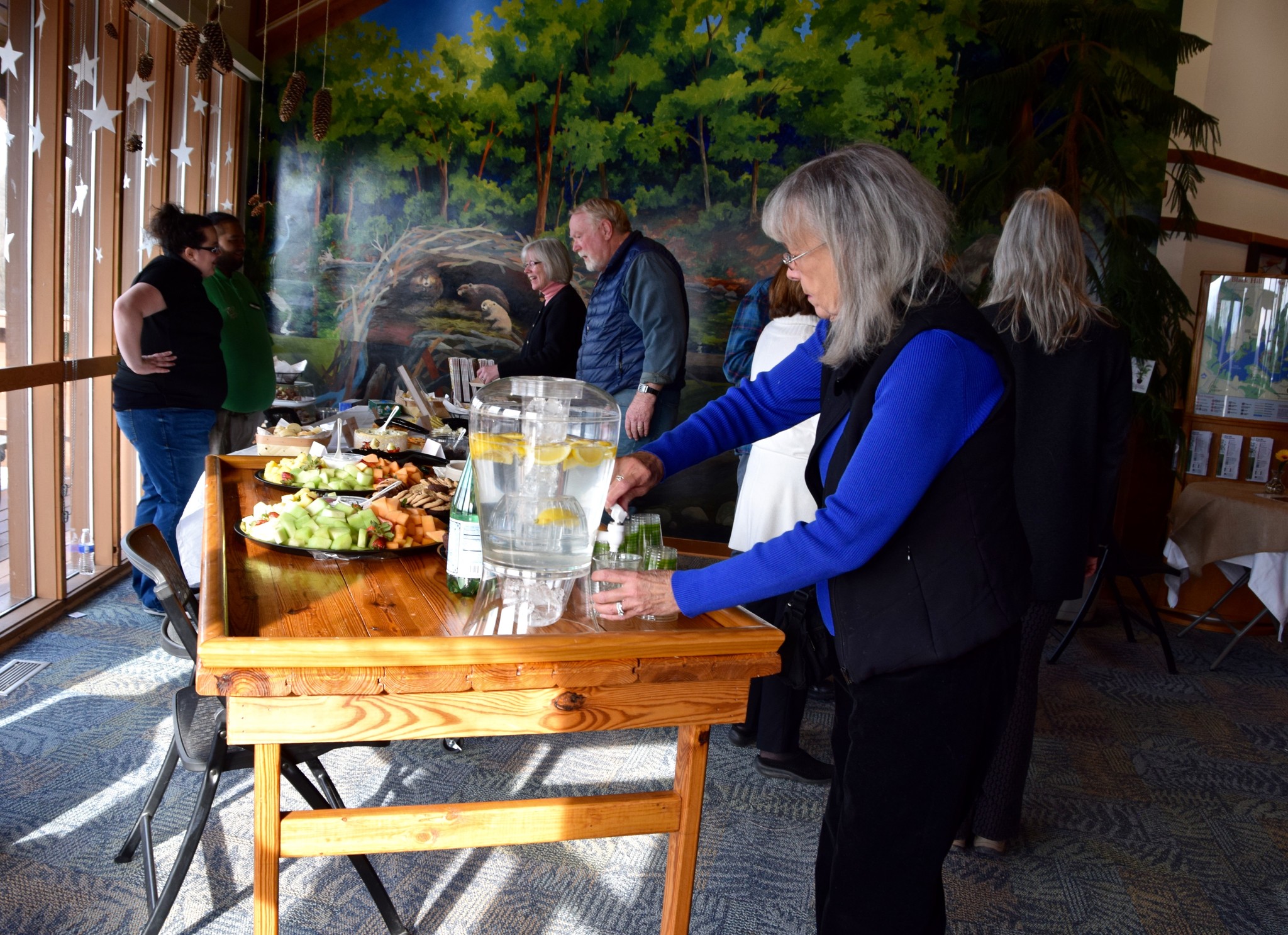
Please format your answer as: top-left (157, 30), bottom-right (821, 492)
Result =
top-left (604, 502), bottom-right (629, 554)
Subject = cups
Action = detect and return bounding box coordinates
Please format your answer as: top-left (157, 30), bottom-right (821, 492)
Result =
top-left (595, 512), bottom-right (678, 623)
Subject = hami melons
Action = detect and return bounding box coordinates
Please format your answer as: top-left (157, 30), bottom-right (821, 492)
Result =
top-left (371, 497), bottom-right (447, 549)
top-left (363, 453), bottom-right (423, 488)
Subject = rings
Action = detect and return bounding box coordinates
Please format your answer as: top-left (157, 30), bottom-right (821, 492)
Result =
top-left (482, 378), bottom-right (485, 381)
top-left (616, 601), bottom-right (624, 616)
top-left (637, 427), bottom-right (643, 429)
top-left (615, 475), bottom-right (623, 481)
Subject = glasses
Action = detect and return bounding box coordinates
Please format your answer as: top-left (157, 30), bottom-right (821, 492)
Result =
top-left (782, 238), bottom-right (830, 271)
top-left (190, 246), bottom-right (221, 254)
top-left (524, 260), bottom-right (542, 269)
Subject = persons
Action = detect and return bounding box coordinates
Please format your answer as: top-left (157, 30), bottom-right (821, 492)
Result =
top-left (111, 204), bottom-right (227, 622)
top-left (476, 238), bottom-right (587, 385)
top-left (585, 145), bottom-right (1016, 935)
top-left (203, 212), bottom-right (277, 457)
top-left (722, 188), bottom-right (1129, 854)
top-left (568, 198), bottom-right (689, 527)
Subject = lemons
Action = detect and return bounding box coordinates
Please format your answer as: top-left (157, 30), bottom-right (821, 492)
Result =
top-left (536, 508), bottom-right (579, 526)
top-left (469, 430), bottom-right (619, 470)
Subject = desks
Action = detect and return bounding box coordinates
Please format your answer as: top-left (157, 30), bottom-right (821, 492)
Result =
top-left (1163, 479), bottom-right (1288, 672)
top-left (177, 404), bottom-right (476, 588)
top-left (197, 449), bottom-right (785, 935)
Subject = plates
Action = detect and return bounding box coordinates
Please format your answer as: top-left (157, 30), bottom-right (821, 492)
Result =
top-left (272, 397), bottom-right (316, 406)
top-left (253, 469), bottom-right (377, 495)
top-left (235, 516), bottom-right (450, 559)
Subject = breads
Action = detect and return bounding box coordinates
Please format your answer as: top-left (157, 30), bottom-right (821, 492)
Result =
top-left (353, 428), bottom-right (408, 452)
top-left (273, 423), bottom-right (322, 437)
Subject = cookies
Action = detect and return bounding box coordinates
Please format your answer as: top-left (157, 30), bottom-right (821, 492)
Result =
top-left (372, 476), bottom-right (459, 511)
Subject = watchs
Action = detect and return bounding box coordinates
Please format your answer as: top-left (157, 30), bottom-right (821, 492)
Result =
top-left (637, 383), bottom-right (660, 397)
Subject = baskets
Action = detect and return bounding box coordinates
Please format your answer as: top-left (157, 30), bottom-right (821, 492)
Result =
top-left (274, 373), bottom-right (302, 384)
top-left (447, 402), bottom-right (470, 420)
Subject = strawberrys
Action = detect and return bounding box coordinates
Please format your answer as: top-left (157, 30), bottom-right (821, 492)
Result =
top-left (282, 472), bottom-right (294, 485)
top-left (383, 474), bottom-right (393, 479)
top-left (352, 501), bottom-right (363, 511)
top-left (251, 510), bottom-right (280, 525)
top-left (376, 478), bottom-right (388, 483)
top-left (316, 465), bottom-right (323, 469)
top-left (309, 496), bottom-right (337, 503)
top-left (366, 521), bottom-right (394, 548)
top-left (359, 459), bottom-right (382, 469)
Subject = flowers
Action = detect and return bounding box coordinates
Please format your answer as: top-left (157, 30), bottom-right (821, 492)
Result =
top-left (1275, 449), bottom-right (1288, 472)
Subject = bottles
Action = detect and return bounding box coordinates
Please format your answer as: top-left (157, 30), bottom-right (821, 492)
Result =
top-left (79, 528), bottom-right (95, 574)
top-left (70, 528), bottom-right (79, 571)
top-left (446, 453), bottom-right (494, 596)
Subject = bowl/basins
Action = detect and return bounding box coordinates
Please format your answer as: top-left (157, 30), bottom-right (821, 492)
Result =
top-left (336, 496), bottom-right (372, 510)
top-left (446, 461), bottom-right (466, 480)
top-left (324, 453), bottom-right (363, 469)
top-left (426, 433), bottom-right (468, 460)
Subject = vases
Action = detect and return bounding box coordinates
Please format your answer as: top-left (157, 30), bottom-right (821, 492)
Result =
top-left (1264, 469), bottom-right (1285, 494)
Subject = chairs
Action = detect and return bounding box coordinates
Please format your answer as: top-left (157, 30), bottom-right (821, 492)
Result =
top-left (1045, 476), bottom-right (1183, 676)
top-left (263, 407), bottom-right (302, 428)
top-left (113, 522), bottom-right (409, 934)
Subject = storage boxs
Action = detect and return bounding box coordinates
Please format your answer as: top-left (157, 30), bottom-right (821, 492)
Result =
top-left (369, 399), bottom-right (400, 418)
top-left (395, 394), bottom-right (449, 418)
top-left (338, 399), bottom-right (363, 411)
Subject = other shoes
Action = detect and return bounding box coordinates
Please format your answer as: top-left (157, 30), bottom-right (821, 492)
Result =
top-left (142, 603), bottom-right (193, 619)
top-left (973, 834), bottom-right (1004, 855)
top-left (729, 725), bottom-right (756, 747)
top-left (949, 839), bottom-right (965, 851)
top-left (754, 749), bottom-right (835, 784)
